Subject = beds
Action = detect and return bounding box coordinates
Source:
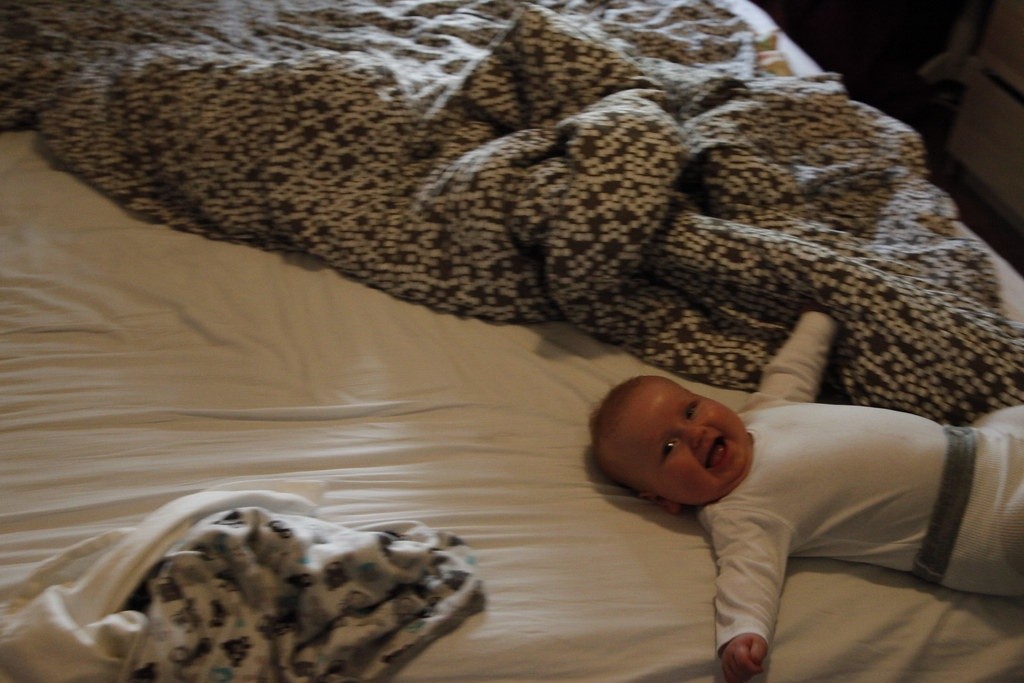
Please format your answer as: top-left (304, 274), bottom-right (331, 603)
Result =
top-left (1, 0), bottom-right (1023, 683)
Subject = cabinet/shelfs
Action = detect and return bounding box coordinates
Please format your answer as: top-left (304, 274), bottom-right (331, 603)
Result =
top-left (944, 0), bottom-right (1024, 236)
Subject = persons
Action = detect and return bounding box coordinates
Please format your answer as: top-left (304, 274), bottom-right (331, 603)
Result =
top-left (589, 298), bottom-right (1024, 683)
